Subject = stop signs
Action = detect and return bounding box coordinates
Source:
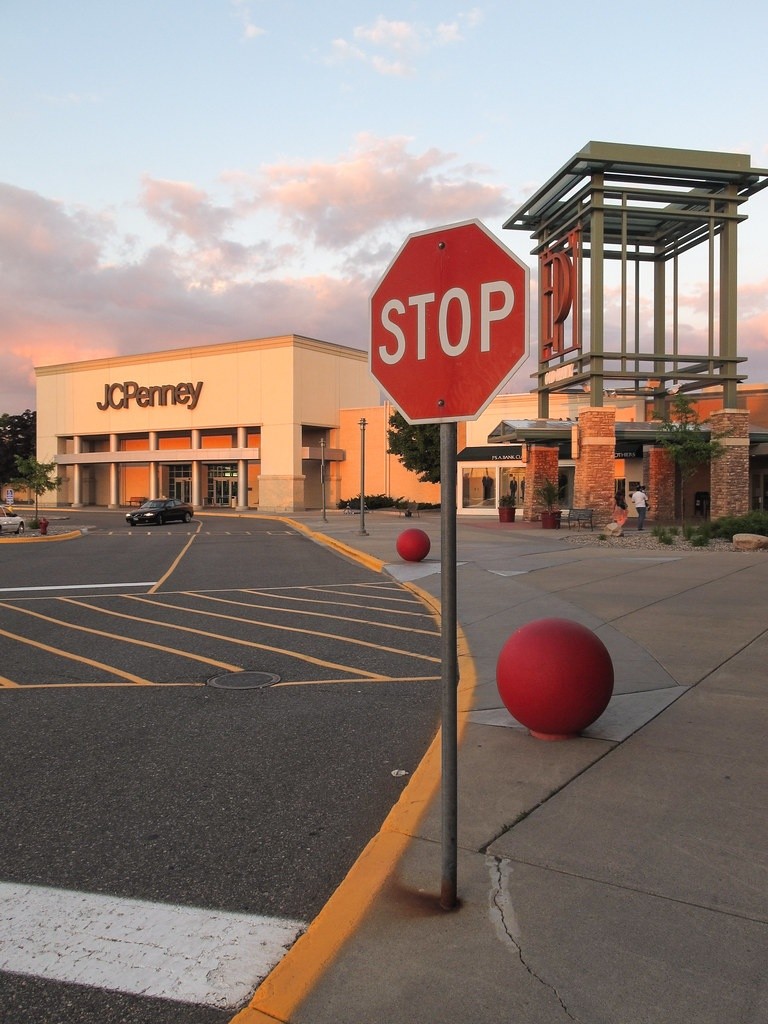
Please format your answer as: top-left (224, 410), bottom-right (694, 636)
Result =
top-left (368, 218), bottom-right (532, 425)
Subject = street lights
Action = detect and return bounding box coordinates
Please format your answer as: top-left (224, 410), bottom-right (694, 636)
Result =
top-left (319, 437), bottom-right (329, 522)
top-left (358, 417), bottom-right (369, 537)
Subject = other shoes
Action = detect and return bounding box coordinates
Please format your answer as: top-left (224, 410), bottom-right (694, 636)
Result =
top-left (638, 528), bottom-right (644, 531)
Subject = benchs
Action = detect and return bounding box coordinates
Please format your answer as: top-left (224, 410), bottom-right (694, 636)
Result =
top-left (556, 509), bottom-right (593, 532)
top-left (125, 497), bottom-right (146, 507)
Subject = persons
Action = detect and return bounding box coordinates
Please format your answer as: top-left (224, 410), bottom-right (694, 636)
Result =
top-left (482, 475), bottom-right (493, 500)
top-left (632, 485), bottom-right (648, 531)
top-left (510, 476), bottom-right (517, 499)
top-left (612, 491), bottom-right (629, 527)
top-left (521, 477), bottom-right (525, 499)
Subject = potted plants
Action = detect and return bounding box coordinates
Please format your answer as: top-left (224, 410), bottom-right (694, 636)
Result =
top-left (497, 494), bottom-right (517, 522)
top-left (536, 478), bottom-right (568, 529)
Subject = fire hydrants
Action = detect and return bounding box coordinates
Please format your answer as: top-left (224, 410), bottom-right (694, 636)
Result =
top-left (40, 517), bottom-right (51, 535)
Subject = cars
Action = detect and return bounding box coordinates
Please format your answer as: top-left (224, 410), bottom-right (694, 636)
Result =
top-left (0, 506), bottom-right (25, 535)
top-left (125, 497), bottom-right (194, 527)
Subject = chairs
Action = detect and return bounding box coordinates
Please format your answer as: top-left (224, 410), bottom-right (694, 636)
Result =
top-left (400, 503), bottom-right (420, 518)
